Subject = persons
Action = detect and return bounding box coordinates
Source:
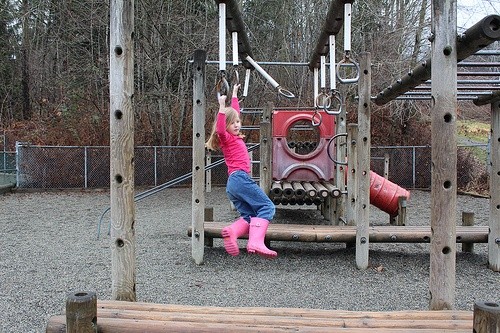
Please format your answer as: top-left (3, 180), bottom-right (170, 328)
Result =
top-left (203, 84), bottom-right (278, 257)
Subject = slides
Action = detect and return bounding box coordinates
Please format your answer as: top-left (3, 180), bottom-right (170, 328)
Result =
top-left (340, 158), bottom-right (412, 216)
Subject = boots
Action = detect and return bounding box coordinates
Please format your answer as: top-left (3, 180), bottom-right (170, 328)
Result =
top-left (221, 216), bottom-right (250, 257)
top-left (246, 215), bottom-right (277, 258)
